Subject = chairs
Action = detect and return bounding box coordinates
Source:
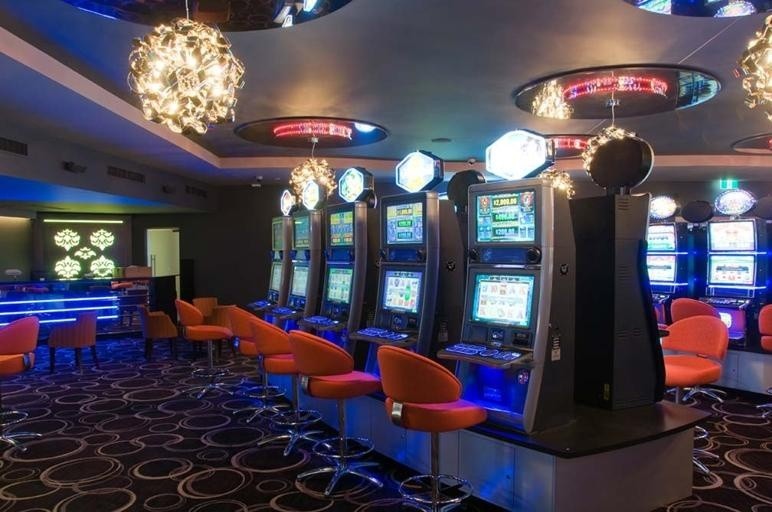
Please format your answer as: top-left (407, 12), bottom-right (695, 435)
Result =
top-left (665, 297), bottom-right (727, 405)
top-left (47, 310), bottom-right (101, 373)
top-left (174, 291), bottom-right (238, 380)
top-left (659, 314), bottom-right (729, 474)
top-left (755, 303), bottom-right (771, 419)
top-left (0, 314), bottom-right (41, 378)
top-left (249, 317), bottom-right (338, 458)
top-left (134, 302), bottom-right (179, 360)
top-left (231, 306), bottom-right (296, 427)
top-left (289, 327), bottom-right (385, 497)
top-left (375, 345), bottom-right (491, 511)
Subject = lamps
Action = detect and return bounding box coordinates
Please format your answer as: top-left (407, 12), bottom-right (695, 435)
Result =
top-left (736, 11), bottom-right (772, 123)
top-left (122, 0), bottom-right (247, 135)
top-left (538, 153), bottom-right (579, 202)
top-left (272, 122), bottom-right (353, 198)
top-left (557, 70), bottom-right (681, 193)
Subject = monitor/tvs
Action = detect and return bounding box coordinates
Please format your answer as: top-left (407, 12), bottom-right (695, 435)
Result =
top-left (326, 268), bottom-right (354, 304)
top-left (709, 222), bottom-right (754, 251)
top-left (645, 255), bottom-right (676, 282)
top-left (381, 271), bottom-right (423, 314)
top-left (386, 202), bottom-right (423, 245)
top-left (289, 264), bottom-right (311, 298)
top-left (329, 208), bottom-right (355, 247)
top-left (291, 213), bottom-right (312, 251)
top-left (471, 275), bottom-right (535, 330)
top-left (646, 224), bottom-right (675, 251)
top-left (272, 220), bottom-right (283, 251)
top-left (709, 255), bottom-right (755, 284)
top-left (269, 262), bottom-right (282, 292)
top-left (476, 189), bottom-right (537, 243)
